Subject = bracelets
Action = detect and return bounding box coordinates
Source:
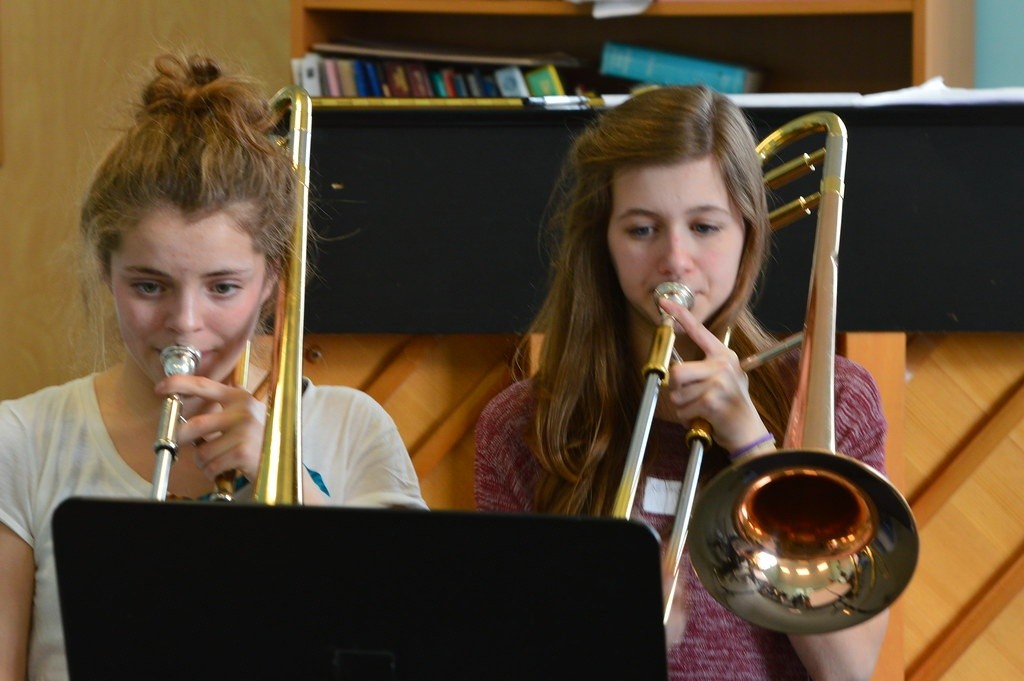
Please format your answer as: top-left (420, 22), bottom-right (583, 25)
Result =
top-left (729, 433), bottom-right (776, 465)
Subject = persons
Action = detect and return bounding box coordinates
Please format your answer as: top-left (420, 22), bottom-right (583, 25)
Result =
top-left (0, 55), bottom-right (431, 681)
top-left (474, 87), bottom-right (887, 681)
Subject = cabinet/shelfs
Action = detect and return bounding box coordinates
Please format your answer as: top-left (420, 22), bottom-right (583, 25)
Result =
top-left (288, 0), bottom-right (977, 90)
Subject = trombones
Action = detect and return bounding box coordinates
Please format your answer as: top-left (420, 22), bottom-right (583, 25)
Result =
top-left (145, 84), bottom-right (315, 506)
top-left (611, 111), bottom-right (924, 637)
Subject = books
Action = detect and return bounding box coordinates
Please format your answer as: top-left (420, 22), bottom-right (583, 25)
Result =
top-left (289, 45), bottom-right (763, 101)
